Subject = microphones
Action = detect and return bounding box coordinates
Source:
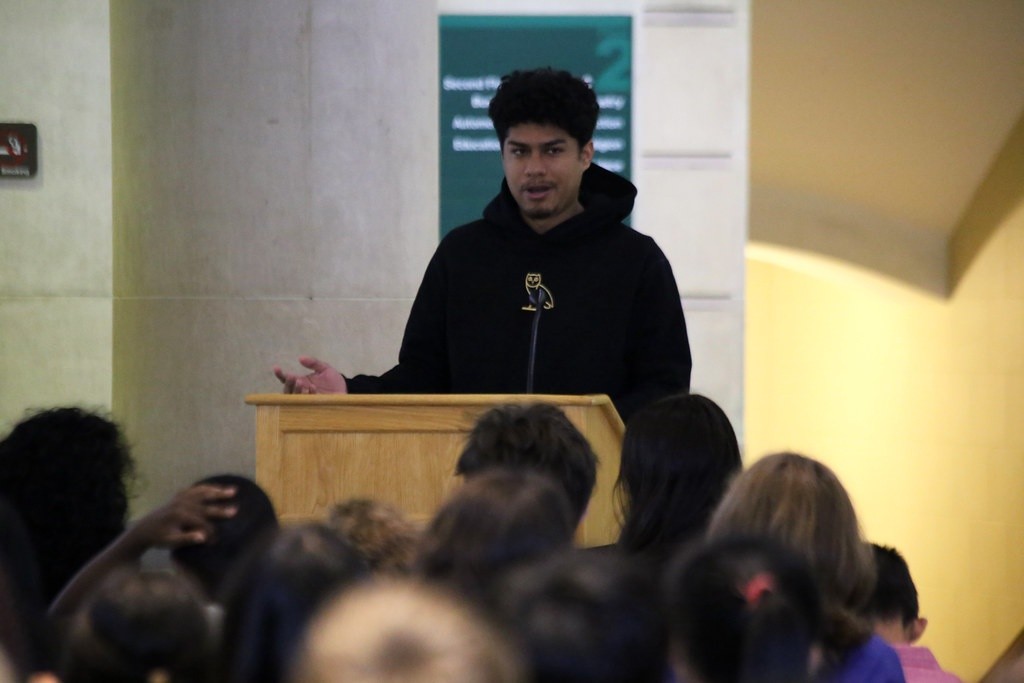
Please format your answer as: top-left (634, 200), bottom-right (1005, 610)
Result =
top-left (526, 279), bottom-right (551, 393)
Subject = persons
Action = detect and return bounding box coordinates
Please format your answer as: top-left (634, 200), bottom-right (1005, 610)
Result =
top-left (275, 66), bottom-right (692, 422)
top-left (0, 393), bottom-right (970, 683)
